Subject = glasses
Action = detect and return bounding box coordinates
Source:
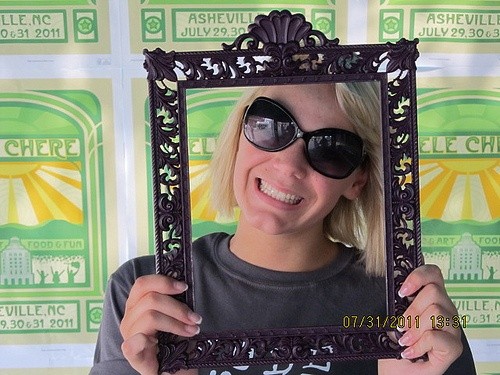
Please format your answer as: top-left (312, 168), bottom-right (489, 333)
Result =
top-left (242, 95), bottom-right (363, 178)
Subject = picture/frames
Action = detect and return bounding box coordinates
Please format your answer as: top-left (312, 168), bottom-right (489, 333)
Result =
top-left (138, 7), bottom-right (430, 375)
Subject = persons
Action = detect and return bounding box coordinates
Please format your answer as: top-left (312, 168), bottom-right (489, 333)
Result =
top-left (87, 37), bottom-right (477, 375)
